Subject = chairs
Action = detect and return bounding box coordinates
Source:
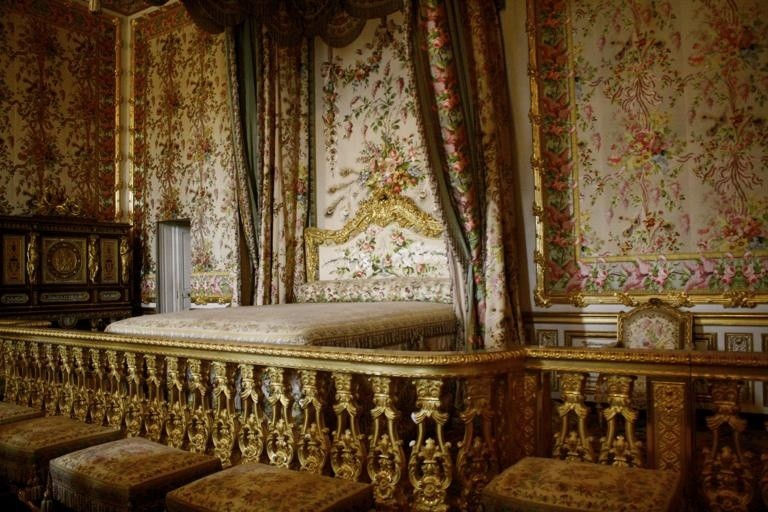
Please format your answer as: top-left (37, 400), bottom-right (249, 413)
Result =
top-left (603, 297), bottom-right (697, 350)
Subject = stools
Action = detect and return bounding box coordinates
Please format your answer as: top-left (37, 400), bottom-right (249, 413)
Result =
top-left (49, 436), bottom-right (221, 512)
top-left (0, 416), bottom-right (122, 512)
top-left (0, 401), bottom-right (41, 424)
top-left (165, 462), bottom-right (374, 512)
top-left (481, 456), bottom-right (680, 512)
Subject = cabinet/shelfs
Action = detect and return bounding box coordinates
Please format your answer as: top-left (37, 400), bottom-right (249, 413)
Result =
top-left (1, 211), bottom-right (134, 332)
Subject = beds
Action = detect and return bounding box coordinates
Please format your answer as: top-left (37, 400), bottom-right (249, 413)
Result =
top-left (104, 192), bottom-right (517, 351)
